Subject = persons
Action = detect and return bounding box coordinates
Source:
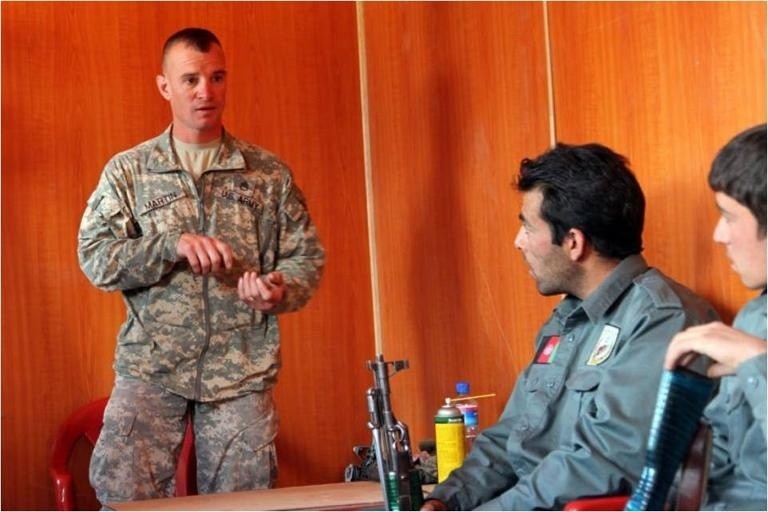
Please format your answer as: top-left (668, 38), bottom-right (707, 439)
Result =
top-left (419, 142), bottom-right (720, 511)
top-left (663, 122), bottom-right (767, 511)
top-left (76, 28), bottom-right (326, 511)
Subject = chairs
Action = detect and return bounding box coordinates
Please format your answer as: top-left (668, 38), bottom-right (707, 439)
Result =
top-left (47, 394), bottom-right (209, 510)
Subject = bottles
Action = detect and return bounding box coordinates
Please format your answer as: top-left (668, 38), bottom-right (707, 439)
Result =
top-left (435, 382), bottom-right (480, 485)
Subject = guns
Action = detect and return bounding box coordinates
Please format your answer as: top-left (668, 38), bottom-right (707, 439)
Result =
top-left (626, 365), bottom-right (714, 510)
top-left (366, 355), bottom-right (425, 510)
